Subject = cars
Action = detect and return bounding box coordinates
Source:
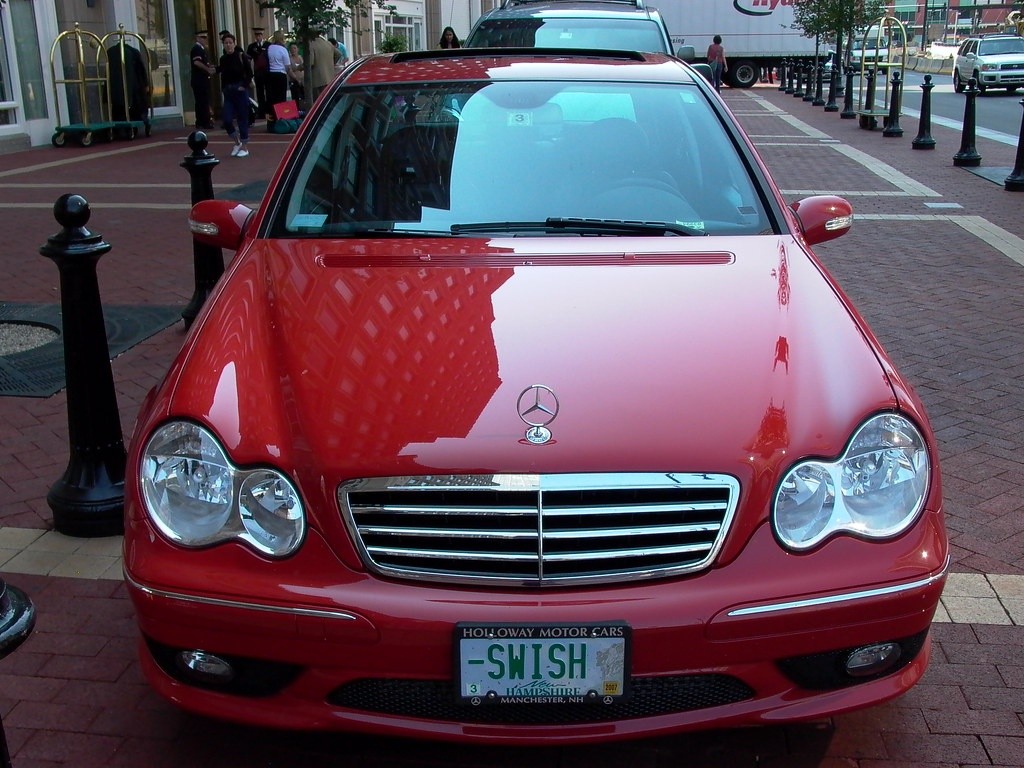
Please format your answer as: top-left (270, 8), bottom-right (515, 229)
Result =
top-left (823, 50), bottom-right (844, 79)
top-left (122, 46), bottom-right (950, 768)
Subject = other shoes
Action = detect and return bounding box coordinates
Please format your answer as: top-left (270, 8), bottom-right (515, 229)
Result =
top-left (237, 149), bottom-right (249, 157)
top-left (196, 122), bottom-right (214, 129)
top-left (231, 143), bottom-right (242, 156)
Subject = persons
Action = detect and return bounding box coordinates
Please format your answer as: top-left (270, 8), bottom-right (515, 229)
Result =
top-left (436, 26), bottom-right (462, 49)
top-left (190, 27), bottom-right (349, 156)
top-left (707, 35), bottom-right (728, 94)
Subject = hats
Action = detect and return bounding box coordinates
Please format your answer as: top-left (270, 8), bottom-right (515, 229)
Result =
top-left (253, 28), bottom-right (265, 34)
top-left (194, 30), bottom-right (209, 37)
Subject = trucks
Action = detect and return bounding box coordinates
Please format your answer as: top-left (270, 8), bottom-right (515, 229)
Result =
top-left (843, 25), bottom-right (888, 75)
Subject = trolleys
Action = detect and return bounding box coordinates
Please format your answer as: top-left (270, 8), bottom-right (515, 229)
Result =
top-left (52, 22), bottom-right (152, 150)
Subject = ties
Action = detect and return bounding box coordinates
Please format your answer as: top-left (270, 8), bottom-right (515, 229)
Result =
top-left (259, 42), bottom-right (261, 51)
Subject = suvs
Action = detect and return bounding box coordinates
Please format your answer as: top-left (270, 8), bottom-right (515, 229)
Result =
top-left (952, 33), bottom-right (1024, 96)
top-left (431, 0), bottom-right (713, 124)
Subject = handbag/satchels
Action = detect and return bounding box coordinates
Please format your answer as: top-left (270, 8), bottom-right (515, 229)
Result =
top-left (273, 100), bottom-right (299, 120)
top-left (273, 118), bottom-right (302, 134)
top-left (239, 52), bottom-right (255, 88)
top-left (710, 60), bottom-right (717, 71)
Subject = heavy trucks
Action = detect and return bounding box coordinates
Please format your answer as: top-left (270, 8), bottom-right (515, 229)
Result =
top-left (637, 0), bottom-right (828, 89)
